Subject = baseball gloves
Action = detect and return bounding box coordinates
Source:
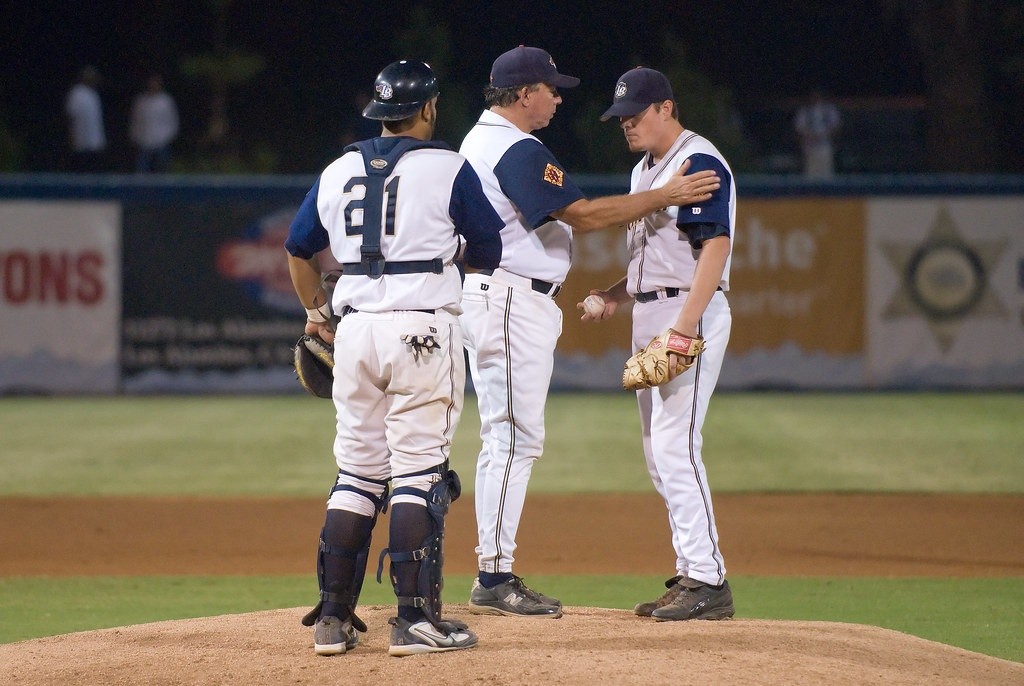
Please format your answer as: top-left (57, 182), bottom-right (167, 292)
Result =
top-left (622, 328), bottom-right (706, 392)
top-left (293, 332), bottom-right (334, 399)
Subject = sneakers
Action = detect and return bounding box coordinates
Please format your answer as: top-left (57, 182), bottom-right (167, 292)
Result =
top-left (634, 576), bottom-right (686, 617)
top-left (651, 576), bottom-right (735, 622)
top-left (471, 576), bottom-right (563, 609)
top-left (469, 577), bottom-right (563, 619)
top-left (388, 615), bottom-right (479, 656)
top-left (313, 616), bottom-right (359, 655)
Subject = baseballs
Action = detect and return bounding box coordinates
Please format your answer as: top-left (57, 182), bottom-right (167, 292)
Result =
top-left (582, 295), bottom-right (607, 318)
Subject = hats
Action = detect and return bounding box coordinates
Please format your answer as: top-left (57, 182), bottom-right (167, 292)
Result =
top-left (490, 45), bottom-right (580, 88)
top-left (600, 66), bottom-right (673, 122)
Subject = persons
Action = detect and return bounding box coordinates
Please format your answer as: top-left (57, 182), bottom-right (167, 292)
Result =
top-left (66, 67), bottom-right (107, 171)
top-left (792, 93), bottom-right (841, 180)
top-left (130, 71), bottom-right (177, 172)
top-left (454, 44), bottom-right (721, 618)
top-left (285, 59), bottom-right (509, 656)
top-left (577, 66), bottom-right (737, 620)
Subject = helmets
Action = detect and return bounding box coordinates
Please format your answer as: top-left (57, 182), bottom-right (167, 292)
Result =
top-left (362, 59), bottom-right (438, 121)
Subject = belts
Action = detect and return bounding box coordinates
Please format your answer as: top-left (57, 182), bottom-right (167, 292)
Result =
top-left (635, 285), bottom-right (722, 303)
top-left (463, 264), bottom-right (562, 300)
top-left (343, 305), bottom-right (435, 315)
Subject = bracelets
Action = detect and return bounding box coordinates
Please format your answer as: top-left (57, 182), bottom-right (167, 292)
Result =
top-left (305, 303), bottom-right (331, 323)
